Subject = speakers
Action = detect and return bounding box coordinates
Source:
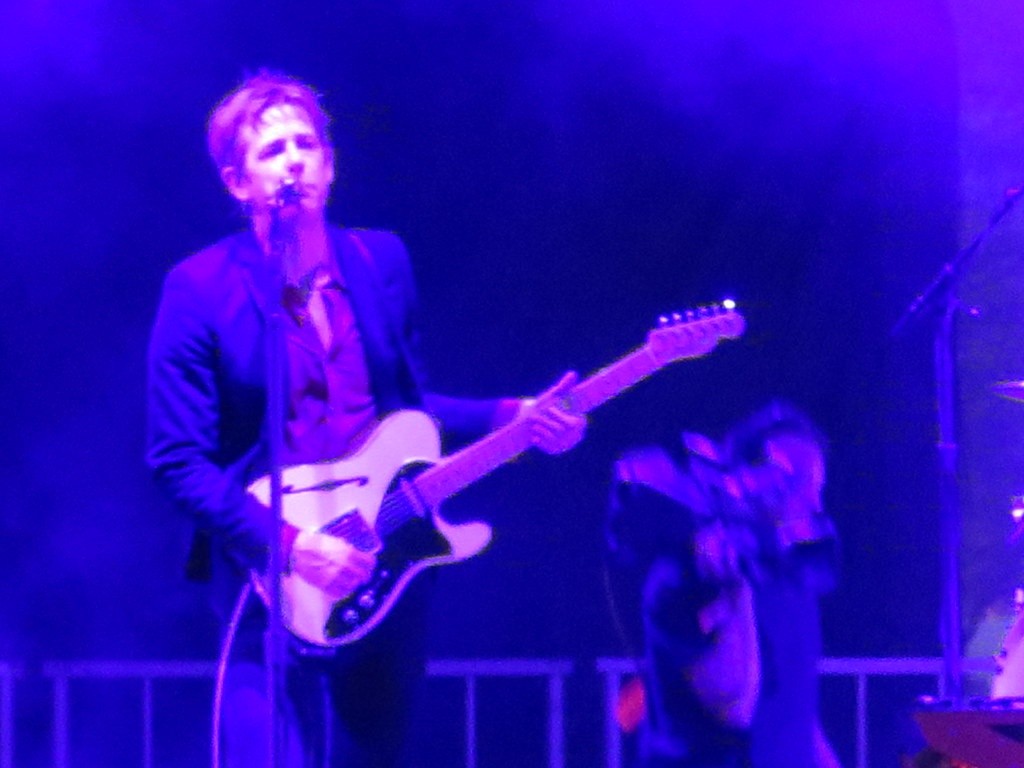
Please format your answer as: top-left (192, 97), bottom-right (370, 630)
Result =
top-left (898, 710), bottom-right (1024, 768)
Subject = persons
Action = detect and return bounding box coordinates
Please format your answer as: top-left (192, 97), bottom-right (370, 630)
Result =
top-left (143, 71), bottom-right (587, 768)
top-left (638, 404), bottom-right (842, 768)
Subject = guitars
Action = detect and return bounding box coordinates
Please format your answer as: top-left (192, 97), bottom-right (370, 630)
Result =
top-left (239, 296), bottom-right (751, 652)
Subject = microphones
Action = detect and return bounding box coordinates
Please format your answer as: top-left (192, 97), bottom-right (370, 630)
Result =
top-left (280, 176), bottom-right (300, 195)
